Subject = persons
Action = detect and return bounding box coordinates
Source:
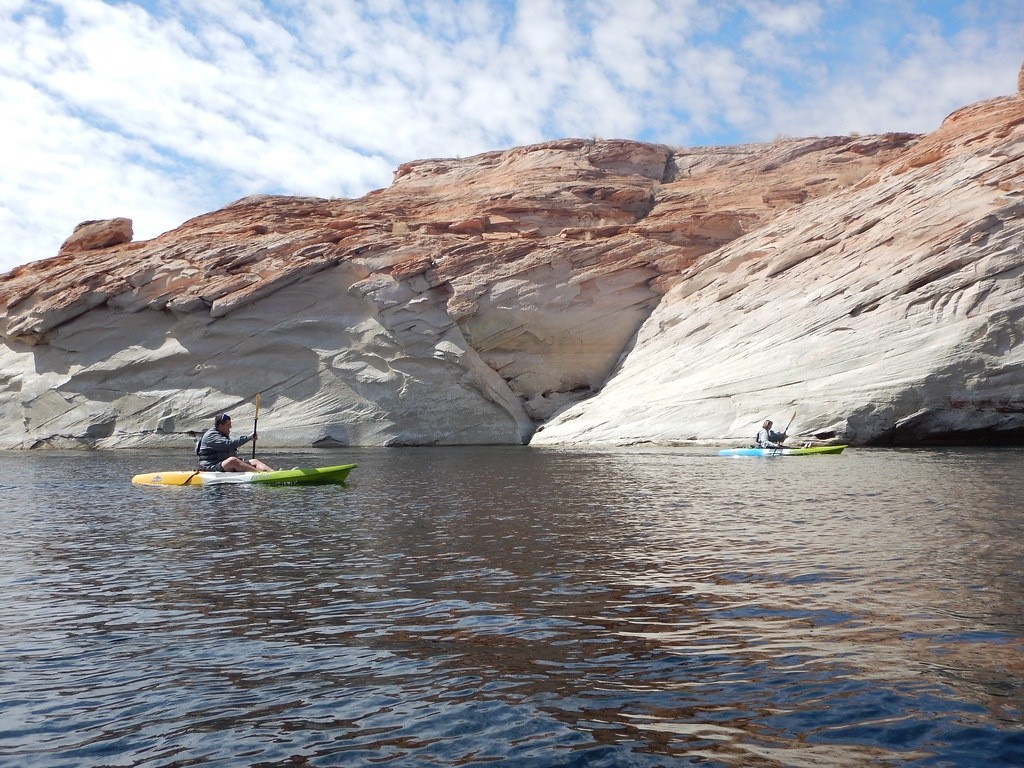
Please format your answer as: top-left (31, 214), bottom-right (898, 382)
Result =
top-left (755, 420), bottom-right (812, 449)
top-left (198, 414), bottom-right (298, 472)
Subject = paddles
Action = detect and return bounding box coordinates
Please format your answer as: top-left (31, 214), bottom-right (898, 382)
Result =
top-left (252, 393), bottom-right (261, 459)
top-left (772, 410), bottom-right (797, 456)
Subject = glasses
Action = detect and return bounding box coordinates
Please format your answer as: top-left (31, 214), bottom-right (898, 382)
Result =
top-left (221, 413), bottom-right (227, 421)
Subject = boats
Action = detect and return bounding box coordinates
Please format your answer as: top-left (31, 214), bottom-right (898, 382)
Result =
top-left (716, 443), bottom-right (849, 457)
top-left (130, 464), bottom-right (363, 487)
